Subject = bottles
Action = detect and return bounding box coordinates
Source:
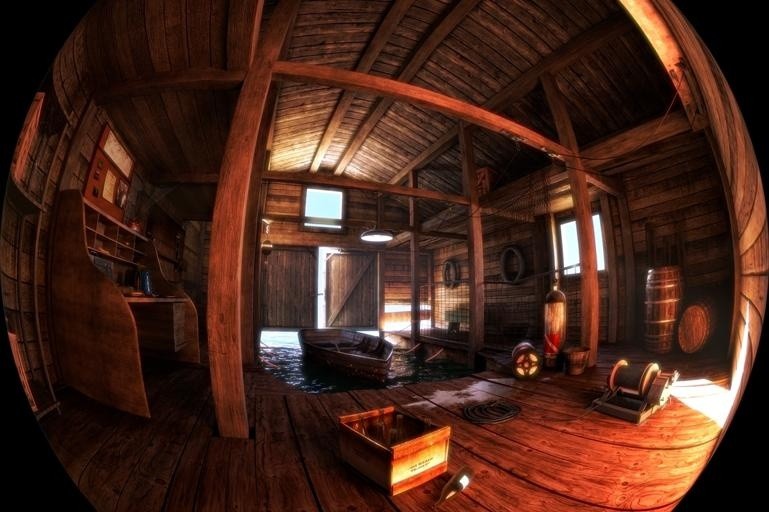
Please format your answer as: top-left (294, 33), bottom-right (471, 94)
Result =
top-left (396, 415), bottom-right (407, 440)
top-left (511, 341), bottom-right (540, 377)
top-left (431, 465), bottom-right (474, 509)
top-left (374, 409), bottom-right (386, 437)
top-left (386, 430), bottom-right (398, 444)
top-left (543, 269), bottom-right (567, 368)
top-left (358, 415), bottom-right (370, 438)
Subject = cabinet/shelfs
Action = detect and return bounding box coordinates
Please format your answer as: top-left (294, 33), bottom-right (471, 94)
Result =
top-left (46, 188), bottom-right (201, 419)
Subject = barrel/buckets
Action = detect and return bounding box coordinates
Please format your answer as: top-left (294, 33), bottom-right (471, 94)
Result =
top-left (640, 262), bottom-right (682, 356)
top-left (676, 296), bottom-right (718, 355)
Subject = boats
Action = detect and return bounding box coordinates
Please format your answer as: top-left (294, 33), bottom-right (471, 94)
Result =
top-left (296, 324), bottom-right (394, 379)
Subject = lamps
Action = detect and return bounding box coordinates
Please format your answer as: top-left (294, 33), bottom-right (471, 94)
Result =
top-left (360, 193), bottom-right (394, 243)
top-left (261, 219), bottom-right (274, 265)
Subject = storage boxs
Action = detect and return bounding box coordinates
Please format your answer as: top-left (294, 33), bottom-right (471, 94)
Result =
top-left (337, 404), bottom-right (451, 496)
